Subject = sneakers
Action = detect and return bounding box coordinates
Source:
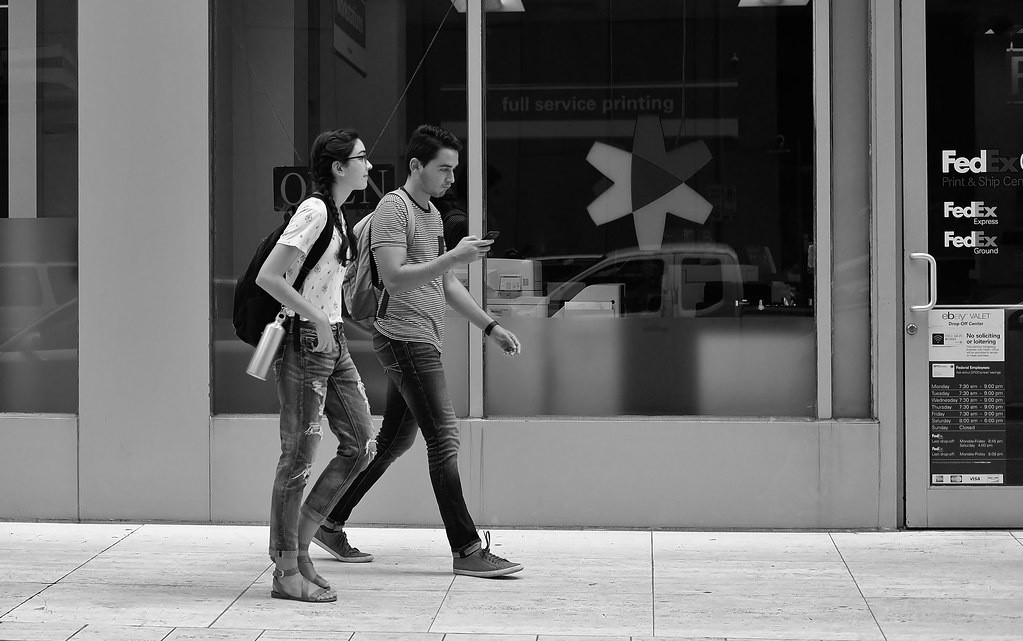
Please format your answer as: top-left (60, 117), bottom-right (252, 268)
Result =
top-left (453, 530), bottom-right (523, 577)
top-left (311, 526), bottom-right (374, 562)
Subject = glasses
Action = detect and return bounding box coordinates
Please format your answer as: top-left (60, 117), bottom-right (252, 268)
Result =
top-left (344, 153), bottom-right (369, 163)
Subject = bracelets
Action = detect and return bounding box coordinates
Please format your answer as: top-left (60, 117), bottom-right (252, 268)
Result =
top-left (483, 320), bottom-right (499, 336)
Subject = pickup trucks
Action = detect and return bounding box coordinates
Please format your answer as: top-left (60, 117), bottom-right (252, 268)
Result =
top-left (521, 243), bottom-right (813, 318)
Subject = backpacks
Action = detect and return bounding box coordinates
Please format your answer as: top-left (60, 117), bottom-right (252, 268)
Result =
top-left (342, 189), bottom-right (416, 323)
top-left (232, 193), bottom-right (336, 355)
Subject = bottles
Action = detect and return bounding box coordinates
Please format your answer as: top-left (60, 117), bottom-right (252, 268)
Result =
top-left (246, 312), bottom-right (286, 381)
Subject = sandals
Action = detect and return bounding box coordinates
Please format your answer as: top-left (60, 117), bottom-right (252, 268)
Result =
top-left (270, 553), bottom-right (339, 603)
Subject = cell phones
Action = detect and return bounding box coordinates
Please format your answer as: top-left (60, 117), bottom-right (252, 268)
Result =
top-left (480, 231), bottom-right (500, 247)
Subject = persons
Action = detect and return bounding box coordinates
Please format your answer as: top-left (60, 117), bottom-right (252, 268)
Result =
top-left (254, 127), bottom-right (377, 602)
top-left (308, 123), bottom-right (527, 576)
